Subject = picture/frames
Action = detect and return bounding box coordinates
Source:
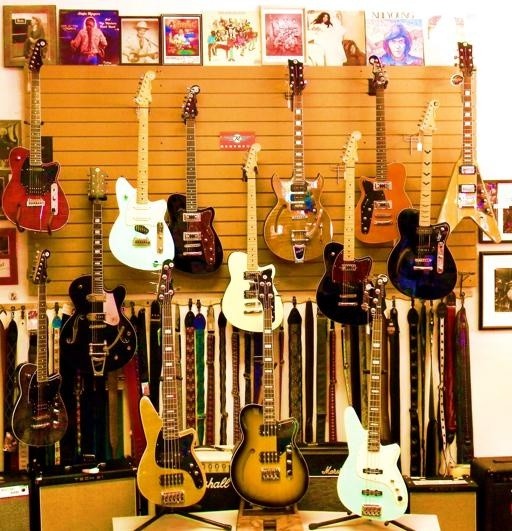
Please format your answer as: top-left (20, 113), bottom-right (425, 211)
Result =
top-left (0, 171), bottom-right (14, 219)
top-left (2, 5), bottom-right (54, 67)
top-left (479, 182), bottom-right (512, 242)
top-left (479, 252), bottom-right (512, 330)
top-left (161, 12), bottom-right (203, 65)
top-left (120, 15), bottom-right (159, 64)
top-left (261, 10), bottom-right (305, 65)
top-left (0, 228), bottom-right (18, 287)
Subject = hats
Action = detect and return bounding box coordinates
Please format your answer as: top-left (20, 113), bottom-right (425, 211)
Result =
top-left (134, 22), bottom-right (151, 30)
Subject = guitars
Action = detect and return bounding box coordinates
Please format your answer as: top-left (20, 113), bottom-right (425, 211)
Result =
top-left (1, 38), bottom-right (69, 233)
top-left (263, 59), bottom-right (333, 265)
top-left (336, 279), bottom-right (409, 522)
top-left (221, 143), bottom-right (283, 334)
top-left (354, 60), bottom-right (414, 243)
top-left (59, 165), bottom-right (137, 377)
top-left (436, 42), bottom-right (503, 244)
top-left (136, 257), bottom-right (207, 508)
top-left (229, 271), bottom-right (310, 508)
top-left (108, 70), bottom-right (175, 273)
top-left (166, 85), bottom-right (223, 275)
top-left (387, 99), bottom-right (457, 300)
top-left (11, 249), bottom-right (69, 447)
top-left (315, 131), bottom-right (377, 326)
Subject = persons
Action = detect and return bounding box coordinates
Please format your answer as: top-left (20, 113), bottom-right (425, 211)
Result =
top-left (306, 12), bottom-right (344, 67)
top-left (379, 22), bottom-right (424, 66)
top-left (71, 17), bottom-right (108, 64)
top-left (270, 17), bottom-right (299, 53)
top-left (123, 23), bottom-right (158, 63)
top-left (24, 16), bottom-right (47, 59)
top-left (168, 23), bottom-right (197, 51)
top-left (208, 18), bottom-right (258, 62)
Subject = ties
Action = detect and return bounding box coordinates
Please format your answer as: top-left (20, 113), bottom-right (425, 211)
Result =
top-left (87, 31), bottom-right (92, 51)
